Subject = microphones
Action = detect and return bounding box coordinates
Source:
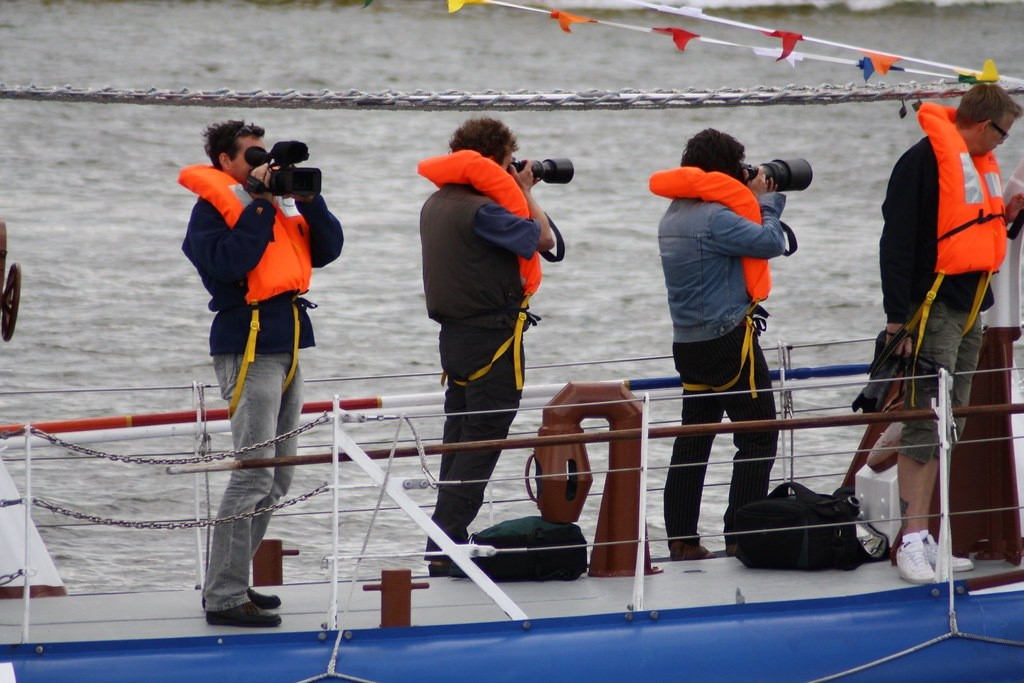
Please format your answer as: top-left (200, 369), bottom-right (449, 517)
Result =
top-left (269, 140), bottom-right (309, 166)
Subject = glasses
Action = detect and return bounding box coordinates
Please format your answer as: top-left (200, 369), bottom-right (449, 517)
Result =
top-left (976, 119), bottom-right (1009, 141)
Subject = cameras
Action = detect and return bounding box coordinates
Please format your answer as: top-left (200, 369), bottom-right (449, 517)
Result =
top-left (508, 157), bottom-right (574, 184)
top-left (739, 158), bottom-right (813, 193)
top-left (852, 330), bottom-right (915, 414)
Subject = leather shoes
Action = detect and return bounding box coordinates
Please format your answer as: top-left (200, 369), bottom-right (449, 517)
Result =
top-left (207, 604), bottom-right (280, 628)
top-left (201, 588), bottom-right (281, 609)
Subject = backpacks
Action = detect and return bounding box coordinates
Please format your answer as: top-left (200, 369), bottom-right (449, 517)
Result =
top-left (451, 516), bottom-right (585, 581)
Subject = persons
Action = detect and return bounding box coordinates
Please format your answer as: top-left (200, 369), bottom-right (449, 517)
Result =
top-left (181, 119), bottom-right (345, 627)
top-left (659, 127), bottom-right (785, 557)
top-left (879, 85), bottom-right (1024, 585)
top-left (417, 116), bottom-right (555, 562)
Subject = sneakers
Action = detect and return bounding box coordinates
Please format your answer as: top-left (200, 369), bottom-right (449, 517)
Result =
top-left (922, 534), bottom-right (975, 574)
top-left (896, 543), bottom-right (935, 584)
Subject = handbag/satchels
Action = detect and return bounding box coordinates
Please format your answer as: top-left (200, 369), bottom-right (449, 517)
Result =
top-left (735, 481), bottom-right (888, 571)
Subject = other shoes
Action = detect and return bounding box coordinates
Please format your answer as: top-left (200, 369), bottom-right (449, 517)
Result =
top-left (427, 558), bottom-right (447, 576)
top-left (669, 542), bottom-right (717, 560)
top-left (724, 542), bottom-right (737, 558)
